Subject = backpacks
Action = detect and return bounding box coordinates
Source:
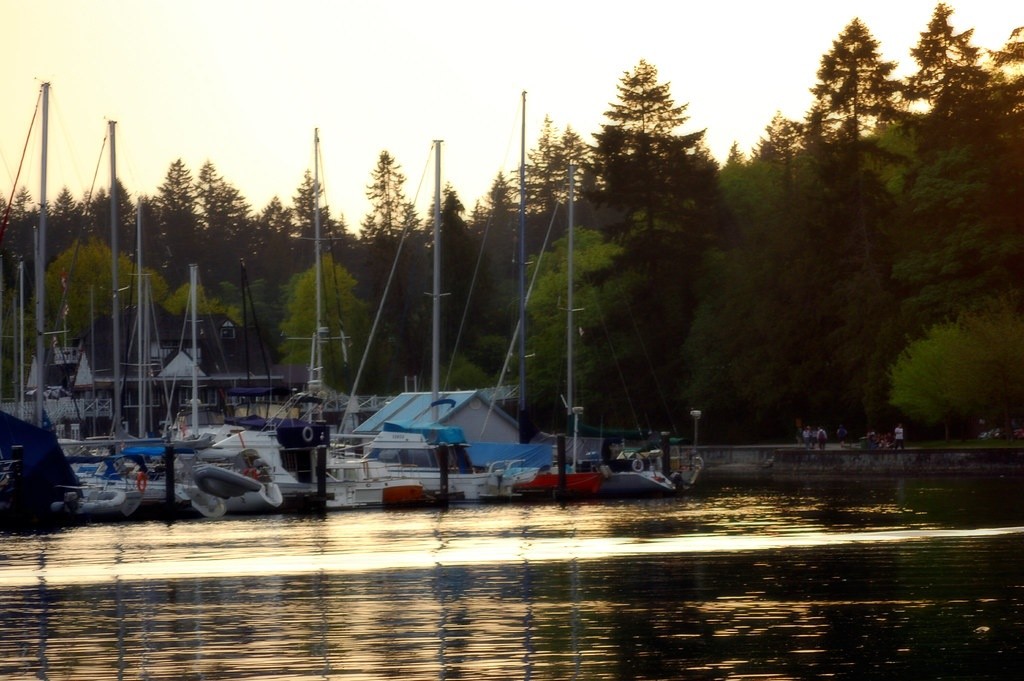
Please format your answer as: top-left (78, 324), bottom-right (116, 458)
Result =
top-left (818, 430), bottom-right (826, 441)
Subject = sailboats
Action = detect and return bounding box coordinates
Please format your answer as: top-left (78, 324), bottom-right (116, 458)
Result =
top-left (0, 81), bottom-right (677, 519)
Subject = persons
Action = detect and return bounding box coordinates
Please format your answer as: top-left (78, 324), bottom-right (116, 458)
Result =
top-left (802, 422), bottom-right (905, 451)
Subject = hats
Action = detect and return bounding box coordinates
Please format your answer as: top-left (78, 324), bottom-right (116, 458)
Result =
top-left (806, 426), bottom-right (810, 429)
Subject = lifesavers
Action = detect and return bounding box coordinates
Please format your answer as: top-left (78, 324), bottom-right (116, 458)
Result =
top-left (302, 426), bottom-right (314, 442)
top-left (632, 459), bottom-right (645, 473)
top-left (136, 471), bottom-right (147, 492)
